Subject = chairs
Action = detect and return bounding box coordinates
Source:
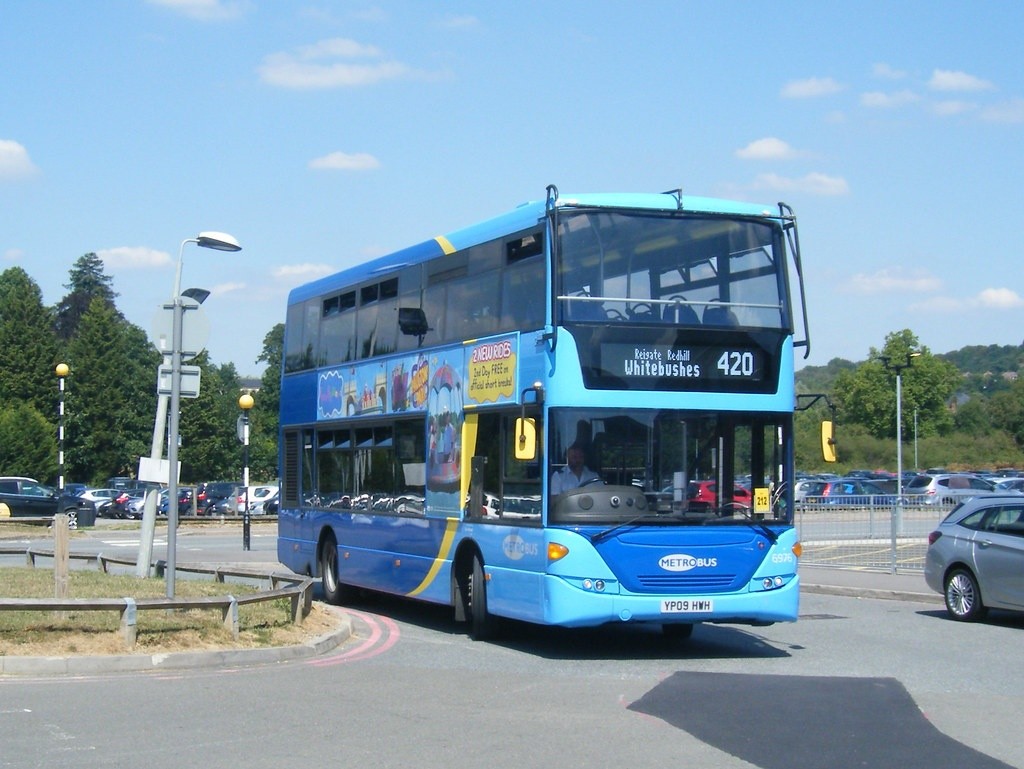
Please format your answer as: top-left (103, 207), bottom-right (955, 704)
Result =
top-left (574, 291), bottom-right (741, 327)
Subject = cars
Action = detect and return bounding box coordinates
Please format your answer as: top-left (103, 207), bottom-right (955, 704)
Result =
top-left (22, 477), bottom-right (279, 520)
top-left (633, 468), bottom-right (1024, 511)
top-left (302, 493), bottom-right (543, 520)
top-left (924, 495), bottom-right (1024, 622)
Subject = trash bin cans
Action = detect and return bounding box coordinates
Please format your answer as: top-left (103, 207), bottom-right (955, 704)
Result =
top-left (77, 500), bottom-right (96, 527)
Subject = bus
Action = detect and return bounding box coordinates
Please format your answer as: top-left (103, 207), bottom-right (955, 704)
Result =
top-left (278, 184), bottom-right (837, 642)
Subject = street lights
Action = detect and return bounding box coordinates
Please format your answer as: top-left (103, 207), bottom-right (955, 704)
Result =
top-left (56, 363), bottom-right (70, 514)
top-left (167, 231), bottom-right (243, 599)
top-left (877, 353), bottom-right (922, 537)
top-left (239, 394), bottom-right (254, 550)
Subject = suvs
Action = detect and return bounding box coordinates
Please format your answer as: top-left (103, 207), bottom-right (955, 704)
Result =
top-left (0, 476), bottom-right (97, 530)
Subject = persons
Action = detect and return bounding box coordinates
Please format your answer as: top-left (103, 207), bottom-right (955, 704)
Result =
top-left (549, 447), bottom-right (602, 503)
